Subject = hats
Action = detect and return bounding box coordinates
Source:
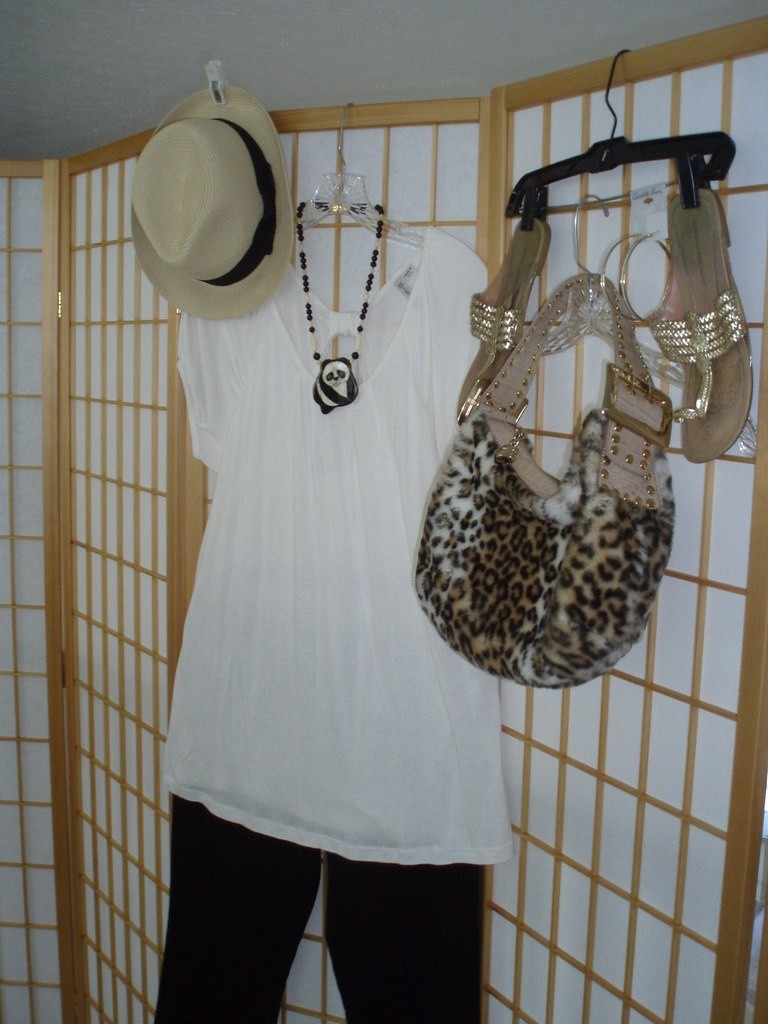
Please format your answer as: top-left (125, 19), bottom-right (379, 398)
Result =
top-left (130, 85), bottom-right (293, 320)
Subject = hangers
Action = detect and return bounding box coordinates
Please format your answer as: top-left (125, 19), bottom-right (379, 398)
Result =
top-left (503, 49), bottom-right (736, 231)
top-left (296, 102), bottom-right (423, 249)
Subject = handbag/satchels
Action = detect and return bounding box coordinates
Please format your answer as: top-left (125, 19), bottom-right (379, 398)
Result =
top-left (413, 271), bottom-right (675, 689)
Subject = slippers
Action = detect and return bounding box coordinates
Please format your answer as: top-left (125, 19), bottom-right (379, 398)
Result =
top-left (454, 217), bottom-right (549, 428)
top-left (650, 190), bottom-right (752, 463)
